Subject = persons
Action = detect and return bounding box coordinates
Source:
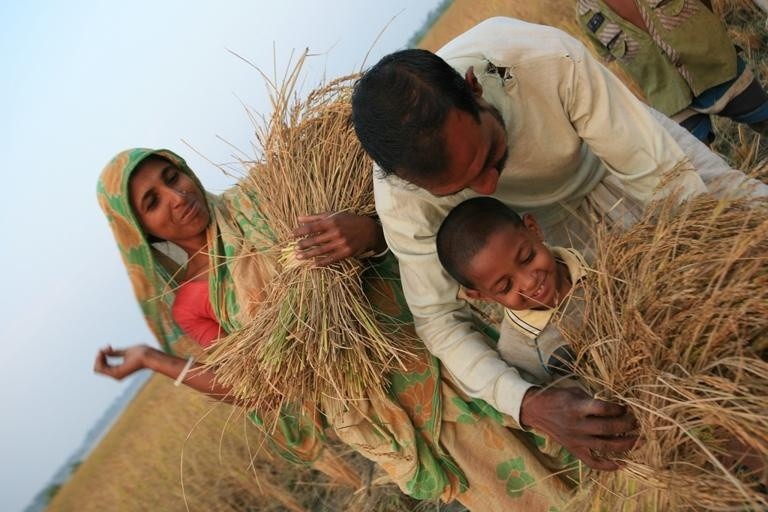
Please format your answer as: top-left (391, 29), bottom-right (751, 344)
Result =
top-left (93, 0), bottom-right (768, 512)
